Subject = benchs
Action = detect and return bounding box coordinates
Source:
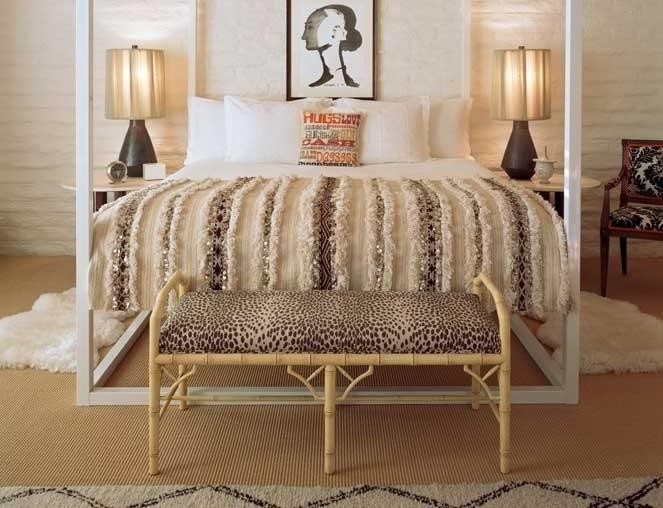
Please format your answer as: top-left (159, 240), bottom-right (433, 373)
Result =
top-left (147, 270), bottom-right (513, 476)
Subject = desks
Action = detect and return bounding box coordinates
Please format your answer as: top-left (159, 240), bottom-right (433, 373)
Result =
top-left (475, 170), bottom-right (605, 218)
top-left (61, 172), bottom-right (159, 216)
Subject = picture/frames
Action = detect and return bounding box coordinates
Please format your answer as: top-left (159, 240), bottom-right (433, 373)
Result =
top-left (285, 0), bottom-right (378, 103)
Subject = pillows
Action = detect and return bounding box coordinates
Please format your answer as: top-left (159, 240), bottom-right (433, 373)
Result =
top-left (297, 108), bottom-right (367, 168)
top-left (334, 94), bottom-right (434, 163)
top-left (183, 93), bottom-right (227, 166)
top-left (222, 94), bottom-right (333, 165)
top-left (427, 96), bottom-right (476, 163)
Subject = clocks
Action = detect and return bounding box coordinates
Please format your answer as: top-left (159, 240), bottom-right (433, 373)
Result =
top-left (105, 161), bottom-right (132, 185)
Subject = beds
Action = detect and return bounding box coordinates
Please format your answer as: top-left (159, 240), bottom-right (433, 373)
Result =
top-left (76, 0), bottom-right (582, 406)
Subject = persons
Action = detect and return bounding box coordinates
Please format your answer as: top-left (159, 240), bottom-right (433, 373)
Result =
top-left (301, 2), bottom-right (364, 89)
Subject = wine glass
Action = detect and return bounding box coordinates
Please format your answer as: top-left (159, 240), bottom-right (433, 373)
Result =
top-left (535, 162), bottom-right (554, 184)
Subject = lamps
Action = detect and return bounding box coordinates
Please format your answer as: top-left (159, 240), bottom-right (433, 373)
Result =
top-left (103, 44), bottom-right (169, 179)
top-left (490, 45), bottom-right (554, 179)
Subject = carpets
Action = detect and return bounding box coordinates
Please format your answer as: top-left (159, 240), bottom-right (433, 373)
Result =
top-left (1, 478), bottom-right (663, 507)
top-left (0, 285), bottom-right (138, 376)
top-left (526, 290), bottom-right (663, 375)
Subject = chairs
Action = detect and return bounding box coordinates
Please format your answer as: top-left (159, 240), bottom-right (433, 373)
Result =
top-left (598, 138), bottom-right (662, 297)
